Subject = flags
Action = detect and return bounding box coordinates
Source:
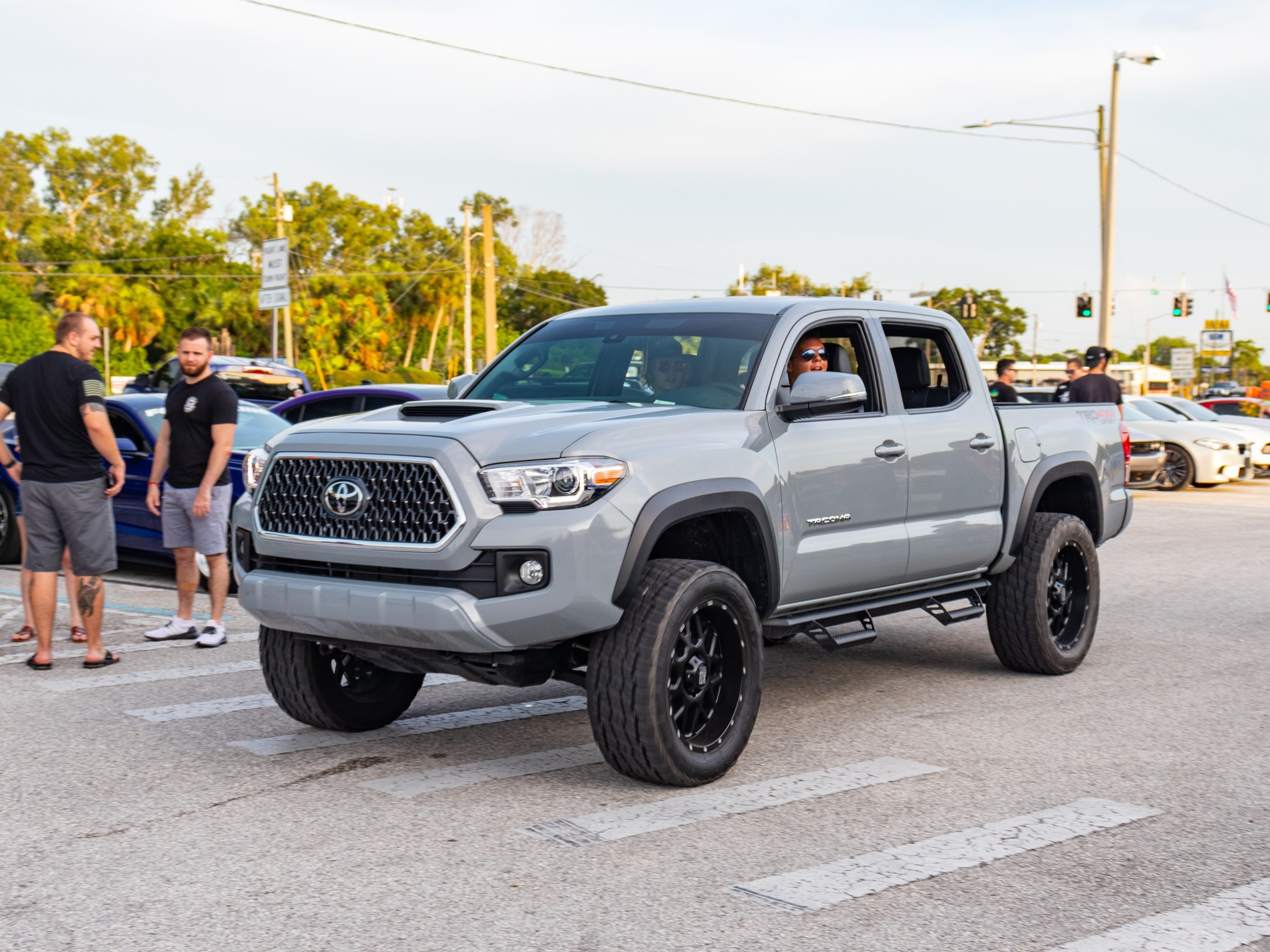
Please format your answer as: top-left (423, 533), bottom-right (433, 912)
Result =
top-left (1223, 271), bottom-right (1239, 321)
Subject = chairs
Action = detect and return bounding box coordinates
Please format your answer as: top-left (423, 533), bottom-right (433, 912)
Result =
top-left (824, 340), bottom-right (866, 415)
top-left (889, 346), bottom-right (951, 409)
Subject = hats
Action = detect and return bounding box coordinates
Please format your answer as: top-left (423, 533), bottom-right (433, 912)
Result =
top-left (1086, 346), bottom-right (1109, 368)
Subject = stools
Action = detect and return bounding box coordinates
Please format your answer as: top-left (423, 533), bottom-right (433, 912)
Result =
top-left (684, 353), bottom-right (708, 390)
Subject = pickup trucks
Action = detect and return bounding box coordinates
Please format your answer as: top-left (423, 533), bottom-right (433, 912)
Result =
top-left (229, 295), bottom-right (1134, 790)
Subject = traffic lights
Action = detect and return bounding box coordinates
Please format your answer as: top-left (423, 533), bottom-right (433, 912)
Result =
top-left (960, 292), bottom-right (977, 319)
top-left (1173, 297), bottom-right (1182, 318)
top-left (1183, 298), bottom-right (1192, 317)
top-left (1266, 293), bottom-right (1270, 313)
top-left (1076, 295), bottom-right (1092, 319)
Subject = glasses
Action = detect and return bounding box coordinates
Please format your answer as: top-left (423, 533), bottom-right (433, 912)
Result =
top-left (1065, 368), bottom-right (1081, 374)
top-left (1006, 368), bottom-right (1017, 373)
top-left (656, 360), bottom-right (685, 372)
top-left (791, 349), bottom-right (830, 362)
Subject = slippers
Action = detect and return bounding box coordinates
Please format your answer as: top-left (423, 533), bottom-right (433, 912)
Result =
top-left (71, 626), bottom-right (88, 643)
top-left (26, 653), bottom-right (53, 671)
top-left (11, 626), bottom-right (38, 642)
top-left (82, 651), bottom-right (120, 669)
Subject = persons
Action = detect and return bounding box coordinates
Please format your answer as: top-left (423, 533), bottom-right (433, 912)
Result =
top-left (1068, 347), bottom-right (1124, 423)
top-left (988, 358), bottom-right (1019, 405)
top-left (0, 314), bottom-right (126, 671)
top-left (7, 412), bottom-right (91, 644)
top-left (641, 333), bottom-right (687, 405)
top-left (145, 327), bottom-right (239, 649)
top-left (787, 335), bottom-right (828, 392)
top-left (1051, 358), bottom-right (1085, 404)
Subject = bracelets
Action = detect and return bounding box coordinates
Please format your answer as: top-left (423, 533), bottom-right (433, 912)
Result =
top-left (146, 482), bottom-right (161, 486)
top-left (2, 459), bottom-right (17, 469)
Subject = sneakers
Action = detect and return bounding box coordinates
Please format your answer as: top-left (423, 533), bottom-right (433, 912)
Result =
top-left (196, 619), bottom-right (227, 646)
top-left (144, 617), bottom-right (199, 641)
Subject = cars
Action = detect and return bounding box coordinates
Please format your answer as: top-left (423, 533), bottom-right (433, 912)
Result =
top-left (1015, 380), bottom-right (1270, 492)
top-left (1, 392), bottom-right (294, 593)
top-left (118, 352), bottom-right (459, 426)
